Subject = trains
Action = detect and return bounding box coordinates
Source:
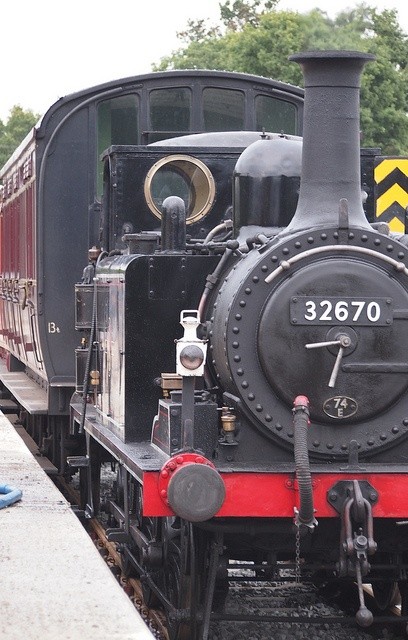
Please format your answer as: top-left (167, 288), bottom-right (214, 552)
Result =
top-left (1, 49), bottom-right (408, 639)
